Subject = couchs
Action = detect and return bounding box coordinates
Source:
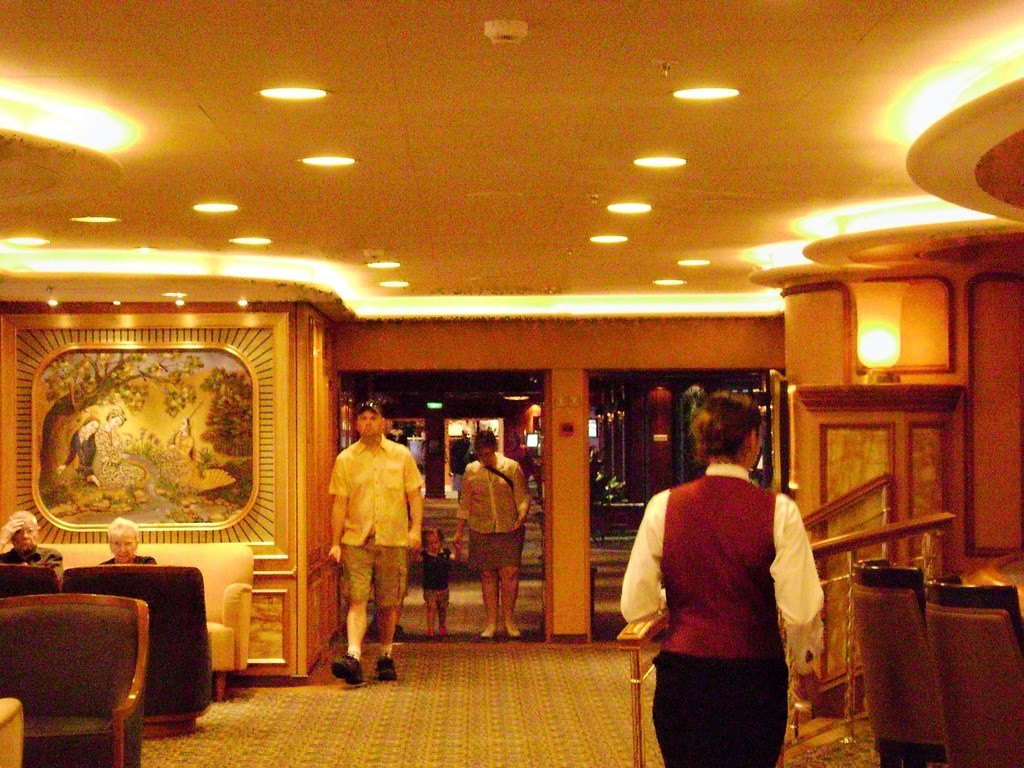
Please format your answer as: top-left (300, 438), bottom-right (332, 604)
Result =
top-left (0, 540), bottom-right (253, 703)
top-left (924, 574), bottom-right (1024, 768)
top-left (0, 563), bottom-right (59, 598)
top-left (0, 593), bottom-right (149, 768)
top-left (64, 561), bottom-right (211, 737)
top-left (851, 558), bottom-right (948, 768)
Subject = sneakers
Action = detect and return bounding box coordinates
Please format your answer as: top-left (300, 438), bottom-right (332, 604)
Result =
top-left (332, 656), bottom-right (364, 684)
top-left (377, 654), bottom-right (396, 680)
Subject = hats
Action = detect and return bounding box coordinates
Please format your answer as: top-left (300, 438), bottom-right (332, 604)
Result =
top-left (357, 401), bottom-right (381, 414)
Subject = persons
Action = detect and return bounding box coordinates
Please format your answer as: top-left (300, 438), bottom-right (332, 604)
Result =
top-left (453, 430), bottom-right (530, 638)
top-left (408, 527), bottom-right (465, 641)
top-left (621, 393), bottom-right (825, 768)
top-left (0, 510), bottom-right (64, 588)
top-left (449, 427), bottom-right (476, 503)
top-left (328, 403), bottom-right (424, 687)
top-left (390, 420), bottom-right (420, 445)
top-left (98, 518), bottom-right (156, 565)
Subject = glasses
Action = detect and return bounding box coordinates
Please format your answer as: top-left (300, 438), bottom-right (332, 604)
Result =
top-left (360, 403), bottom-right (378, 407)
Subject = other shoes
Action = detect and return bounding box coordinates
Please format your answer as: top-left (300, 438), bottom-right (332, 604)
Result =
top-left (481, 625), bottom-right (498, 637)
top-left (426, 627), bottom-right (446, 638)
top-left (505, 622), bottom-right (520, 638)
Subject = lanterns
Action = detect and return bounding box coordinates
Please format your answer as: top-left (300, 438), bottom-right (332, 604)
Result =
top-left (647, 387), bottom-right (672, 410)
